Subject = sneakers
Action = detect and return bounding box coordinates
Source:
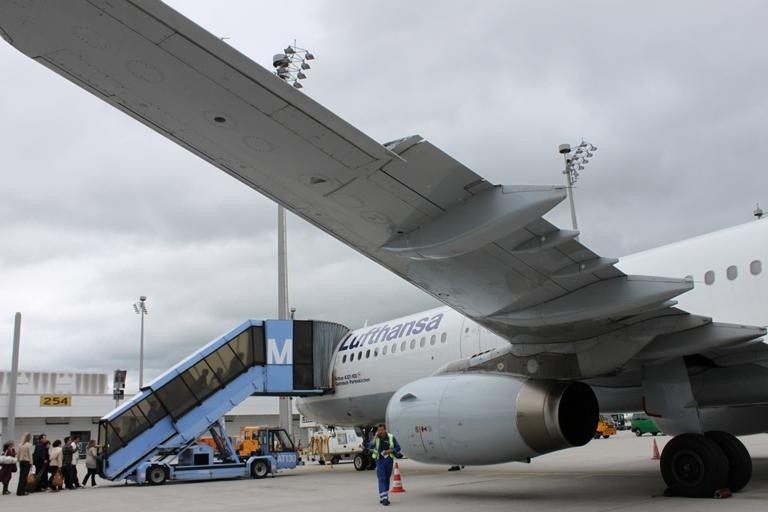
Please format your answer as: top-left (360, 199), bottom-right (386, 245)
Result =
top-left (380, 499), bottom-right (390, 506)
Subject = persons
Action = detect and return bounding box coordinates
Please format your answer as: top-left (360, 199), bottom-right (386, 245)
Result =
top-left (367, 423), bottom-right (402, 506)
top-left (197, 368), bottom-right (209, 388)
top-left (0, 431), bottom-right (105, 496)
top-left (229, 351), bottom-right (246, 378)
top-left (209, 367), bottom-right (227, 391)
top-left (127, 416), bottom-right (146, 441)
top-left (447, 465), bottom-right (460, 471)
top-left (147, 400), bottom-right (161, 422)
top-left (110, 428), bottom-right (122, 442)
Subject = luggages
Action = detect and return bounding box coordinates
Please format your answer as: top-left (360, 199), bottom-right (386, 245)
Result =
top-left (25, 460), bottom-right (51, 492)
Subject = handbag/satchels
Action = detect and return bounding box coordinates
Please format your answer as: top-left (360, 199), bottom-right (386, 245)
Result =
top-left (52, 473), bottom-right (63, 486)
top-left (0, 456), bottom-right (17, 464)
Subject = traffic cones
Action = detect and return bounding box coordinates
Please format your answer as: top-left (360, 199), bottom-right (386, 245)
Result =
top-left (391, 461), bottom-right (405, 493)
top-left (650, 436), bottom-right (662, 461)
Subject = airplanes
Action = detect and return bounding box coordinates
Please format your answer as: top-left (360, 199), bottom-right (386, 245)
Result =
top-left (1, 0), bottom-right (768, 501)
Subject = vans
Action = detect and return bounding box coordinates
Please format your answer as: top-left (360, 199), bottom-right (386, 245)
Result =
top-left (592, 410), bottom-right (666, 439)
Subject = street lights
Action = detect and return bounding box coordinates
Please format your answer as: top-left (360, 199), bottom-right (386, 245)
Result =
top-left (136, 296), bottom-right (149, 392)
top-left (268, 38), bottom-right (318, 440)
top-left (556, 134), bottom-right (602, 243)
